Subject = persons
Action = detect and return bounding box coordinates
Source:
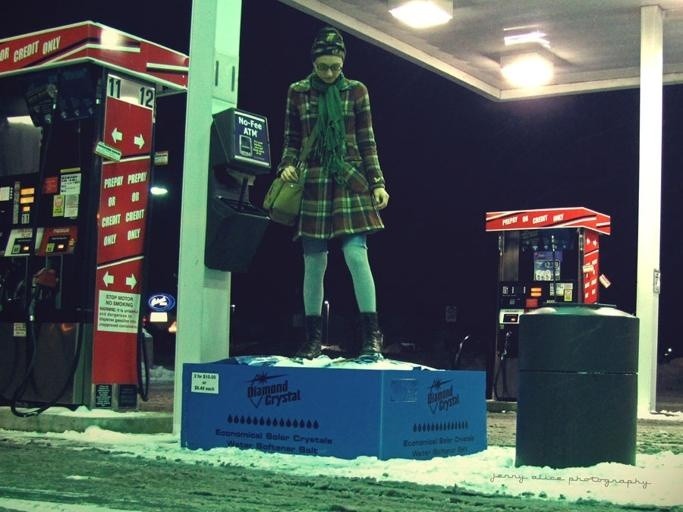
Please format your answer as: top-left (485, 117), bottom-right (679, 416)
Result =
top-left (276, 27), bottom-right (390, 361)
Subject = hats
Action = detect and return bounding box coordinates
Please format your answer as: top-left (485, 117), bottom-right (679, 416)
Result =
top-left (309, 28), bottom-right (346, 63)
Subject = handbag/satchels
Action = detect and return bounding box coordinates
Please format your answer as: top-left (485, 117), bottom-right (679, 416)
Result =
top-left (262, 176), bottom-right (303, 216)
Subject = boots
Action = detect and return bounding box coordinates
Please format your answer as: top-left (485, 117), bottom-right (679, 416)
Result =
top-left (356, 312), bottom-right (384, 363)
top-left (296, 315), bottom-right (323, 359)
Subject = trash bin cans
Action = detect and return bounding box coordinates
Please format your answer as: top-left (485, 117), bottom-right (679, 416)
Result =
top-left (513, 301), bottom-right (640, 471)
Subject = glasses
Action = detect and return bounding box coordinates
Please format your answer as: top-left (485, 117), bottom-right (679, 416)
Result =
top-left (314, 62), bottom-right (342, 72)
